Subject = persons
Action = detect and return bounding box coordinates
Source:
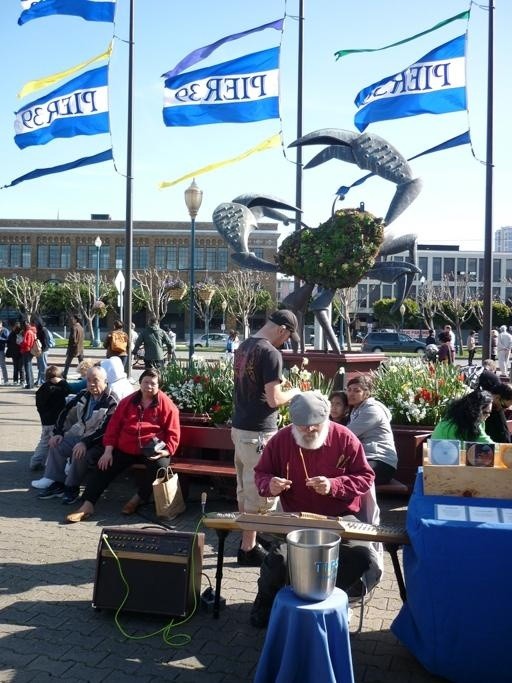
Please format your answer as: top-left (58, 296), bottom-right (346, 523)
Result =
top-left (0, 316), bottom-right (182, 523)
top-left (327, 390), bottom-right (348, 424)
top-left (346, 375), bottom-right (397, 485)
top-left (227, 328), bottom-right (235, 352)
top-left (229, 330), bottom-right (240, 352)
top-left (250, 387), bottom-right (384, 627)
top-left (420, 323), bottom-right (512, 442)
top-left (231, 308), bottom-right (304, 565)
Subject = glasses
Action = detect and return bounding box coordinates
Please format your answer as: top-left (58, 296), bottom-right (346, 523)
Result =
top-left (482, 409), bottom-right (492, 415)
top-left (500, 400), bottom-right (508, 409)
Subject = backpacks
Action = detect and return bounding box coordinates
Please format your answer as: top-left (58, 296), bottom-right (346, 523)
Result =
top-left (111, 331), bottom-right (127, 351)
top-left (29, 330), bottom-right (43, 356)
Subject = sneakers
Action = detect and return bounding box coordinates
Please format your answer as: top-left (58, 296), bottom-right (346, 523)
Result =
top-left (1, 382), bottom-right (43, 388)
top-left (250, 591), bottom-right (274, 628)
top-left (31, 477), bottom-right (55, 489)
top-left (62, 488), bottom-right (81, 505)
top-left (38, 484), bottom-right (65, 499)
top-left (235, 535), bottom-right (285, 566)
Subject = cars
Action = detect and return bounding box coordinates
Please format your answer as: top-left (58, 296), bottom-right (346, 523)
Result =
top-left (474, 333), bottom-right (480, 344)
top-left (185, 332), bottom-right (229, 349)
top-left (355, 333), bottom-right (366, 343)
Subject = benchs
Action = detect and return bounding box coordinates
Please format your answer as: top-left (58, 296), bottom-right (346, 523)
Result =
top-left (172, 424), bottom-right (434, 501)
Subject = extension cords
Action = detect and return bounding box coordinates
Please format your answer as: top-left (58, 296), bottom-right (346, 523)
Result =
top-left (200, 585), bottom-right (226, 612)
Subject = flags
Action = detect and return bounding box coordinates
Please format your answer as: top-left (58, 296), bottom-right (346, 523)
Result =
top-left (13, 62), bottom-right (111, 149)
top-left (353, 31), bottom-right (469, 132)
top-left (162, 44), bottom-right (281, 125)
top-left (17, 0), bottom-right (115, 23)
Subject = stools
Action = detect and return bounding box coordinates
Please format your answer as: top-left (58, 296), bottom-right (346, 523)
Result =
top-left (255, 584), bottom-right (356, 683)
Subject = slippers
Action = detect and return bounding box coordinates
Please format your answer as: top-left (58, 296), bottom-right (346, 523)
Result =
top-left (65, 508), bottom-right (95, 522)
top-left (121, 501), bottom-right (137, 515)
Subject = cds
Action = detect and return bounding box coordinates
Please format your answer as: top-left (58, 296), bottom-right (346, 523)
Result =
top-left (503, 447), bottom-right (512, 468)
top-left (467, 444), bottom-right (493, 466)
top-left (433, 441), bottom-right (458, 465)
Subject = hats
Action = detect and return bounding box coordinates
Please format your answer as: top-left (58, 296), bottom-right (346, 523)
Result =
top-left (499, 325), bottom-right (506, 330)
top-left (289, 390), bottom-right (330, 424)
top-left (267, 308), bottom-right (302, 343)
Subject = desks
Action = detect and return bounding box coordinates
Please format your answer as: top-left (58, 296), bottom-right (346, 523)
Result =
top-left (393, 465), bottom-right (512, 679)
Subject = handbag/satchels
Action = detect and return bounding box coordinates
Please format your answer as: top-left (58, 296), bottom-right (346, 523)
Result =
top-left (152, 465), bottom-right (187, 519)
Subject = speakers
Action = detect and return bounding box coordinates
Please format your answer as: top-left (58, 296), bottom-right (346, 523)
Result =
top-left (93, 527), bottom-right (206, 619)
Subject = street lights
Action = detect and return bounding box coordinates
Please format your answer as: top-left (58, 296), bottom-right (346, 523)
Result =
top-left (202, 299), bottom-right (212, 346)
top-left (457, 270), bottom-right (477, 307)
top-left (92, 235), bottom-right (104, 347)
top-left (185, 179), bottom-right (204, 375)
top-left (398, 303), bottom-right (407, 331)
top-left (418, 276), bottom-right (427, 340)
top-left (220, 300), bottom-right (228, 334)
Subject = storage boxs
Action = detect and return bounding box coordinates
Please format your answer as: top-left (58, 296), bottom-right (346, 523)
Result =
top-left (421, 441), bottom-right (512, 499)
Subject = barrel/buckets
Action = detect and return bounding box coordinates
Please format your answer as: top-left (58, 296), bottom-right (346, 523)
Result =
top-left (285, 528), bottom-right (342, 602)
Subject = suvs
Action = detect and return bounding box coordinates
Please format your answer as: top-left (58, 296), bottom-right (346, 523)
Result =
top-left (359, 332), bottom-right (428, 353)
